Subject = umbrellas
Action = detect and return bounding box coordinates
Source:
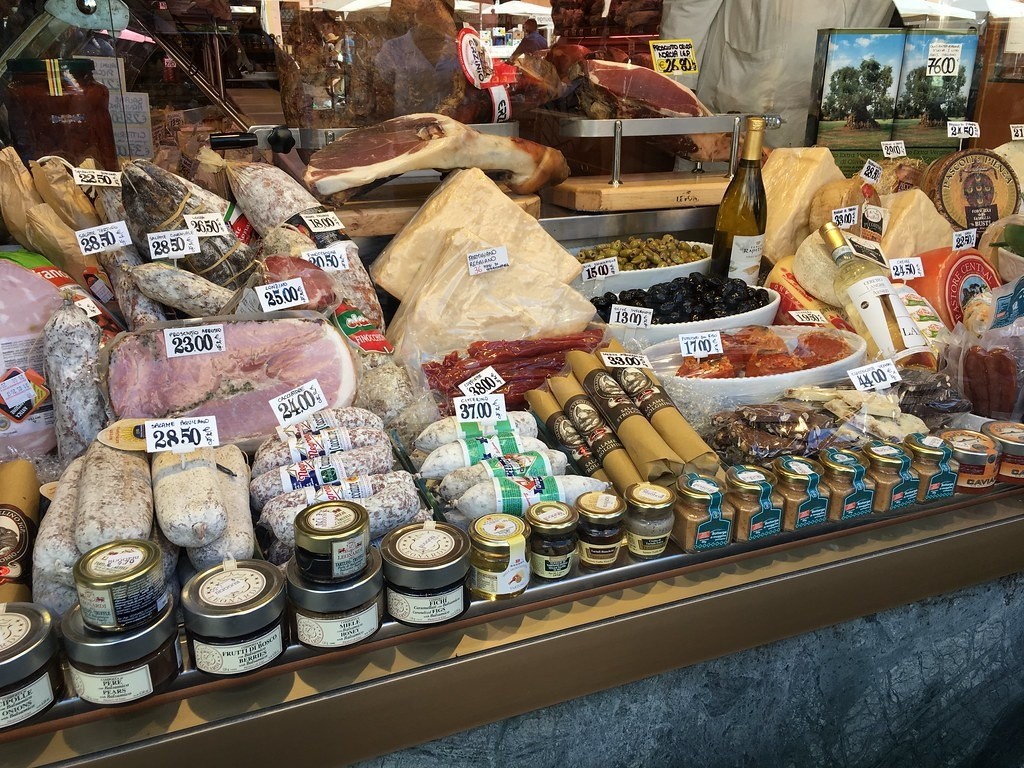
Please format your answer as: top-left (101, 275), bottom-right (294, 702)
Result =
top-left (480, 0), bottom-right (552, 27)
top-left (301, 0), bottom-right (478, 19)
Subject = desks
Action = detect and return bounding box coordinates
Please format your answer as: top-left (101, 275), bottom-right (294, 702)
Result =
top-left (151, 31), bottom-right (234, 101)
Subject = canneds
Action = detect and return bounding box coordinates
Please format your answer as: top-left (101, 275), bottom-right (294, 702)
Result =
top-left (0, 422), bottom-right (1024, 728)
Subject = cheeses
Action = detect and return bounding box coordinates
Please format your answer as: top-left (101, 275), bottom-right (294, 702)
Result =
top-left (369, 141), bottom-right (1024, 396)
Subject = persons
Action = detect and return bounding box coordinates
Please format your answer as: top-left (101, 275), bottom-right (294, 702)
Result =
top-left (510, 19), bottom-right (547, 62)
top-left (331, 20), bottom-right (356, 99)
top-left (372, 0), bottom-right (467, 118)
top-left (658, 0), bottom-right (895, 177)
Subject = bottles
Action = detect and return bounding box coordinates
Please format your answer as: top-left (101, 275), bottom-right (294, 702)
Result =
top-left (710, 118), bottom-right (767, 286)
top-left (818, 221), bottom-right (937, 372)
